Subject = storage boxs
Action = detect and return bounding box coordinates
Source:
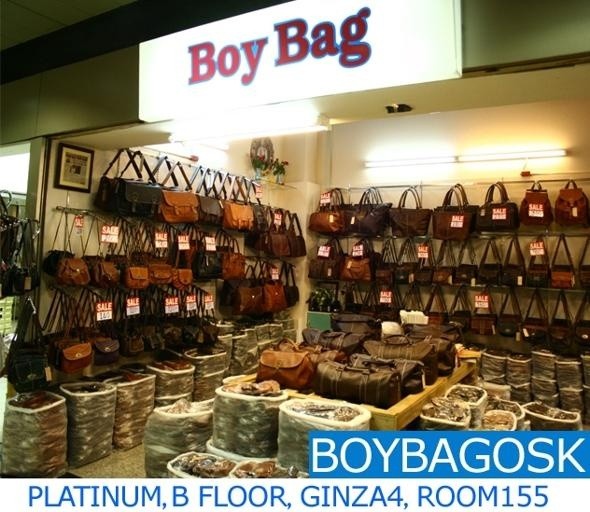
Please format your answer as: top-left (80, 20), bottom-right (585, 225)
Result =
top-left (306, 311), bottom-right (336, 330)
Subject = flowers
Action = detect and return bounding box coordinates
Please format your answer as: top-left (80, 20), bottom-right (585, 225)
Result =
top-left (271, 158), bottom-right (289, 176)
top-left (254, 155), bottom-right (268, 171)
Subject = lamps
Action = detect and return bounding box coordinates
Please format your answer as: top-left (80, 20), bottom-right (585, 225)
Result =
top-left (144, 145), bottom-right (199, 162)
top-left (363, 147), bottom-right (566, 171)
top-left (169, 114), bottom-right (332, 144)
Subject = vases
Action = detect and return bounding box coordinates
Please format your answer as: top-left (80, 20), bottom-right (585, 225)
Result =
top-left (255, 168), bottom-right (262, 180)
top-left (276, 171), bottom-right (285, 185)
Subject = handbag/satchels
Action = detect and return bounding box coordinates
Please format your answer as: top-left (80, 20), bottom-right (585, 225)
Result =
top-left (248, 180), bottom-right (589, 407)
top-left (2, 147), bottom-right (263, 393)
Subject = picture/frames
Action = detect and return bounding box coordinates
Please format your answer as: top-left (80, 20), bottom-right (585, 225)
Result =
top-left (317, 281), bottom-right (339, 303)
top-left (54, 142), bottom-right (94, 193)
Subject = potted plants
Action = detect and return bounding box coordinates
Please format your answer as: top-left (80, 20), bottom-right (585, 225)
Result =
top-left (311, 287), bottom-right (332, 311)
